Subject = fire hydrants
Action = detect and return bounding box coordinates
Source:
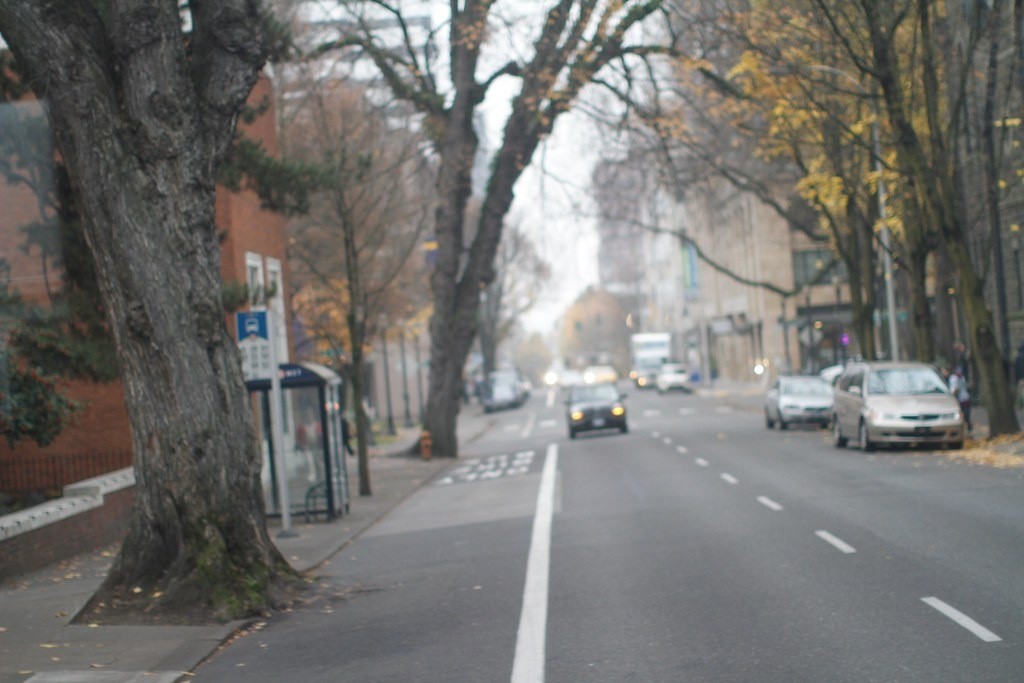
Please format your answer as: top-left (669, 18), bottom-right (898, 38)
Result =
top-left (420, 430), bottom-right (433, 461)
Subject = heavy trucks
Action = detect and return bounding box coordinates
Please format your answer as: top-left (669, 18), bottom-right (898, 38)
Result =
top-left (628, 333), bottom-right (673, 387)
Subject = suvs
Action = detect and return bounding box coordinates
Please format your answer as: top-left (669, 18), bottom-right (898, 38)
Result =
top-left (557, 365), bottom-right (618, 390)
top-left (657, 363), bottom-right (693, 395)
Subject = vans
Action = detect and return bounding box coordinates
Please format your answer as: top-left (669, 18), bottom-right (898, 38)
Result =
top-left (831, 360), bottom-right (965, 453)
top-left (479, 370), bottom-right (523, 412)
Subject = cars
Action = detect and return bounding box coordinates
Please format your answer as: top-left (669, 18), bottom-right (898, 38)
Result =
top-left (564, 379), bottom-right (629, 440)
top-left (764, 374), bottom-right (834, 431)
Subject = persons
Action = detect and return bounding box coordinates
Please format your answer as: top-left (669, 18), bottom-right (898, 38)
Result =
top-left (297, 407), bottom-right (322, 482)
top-left (940, 339), bottom-right (973, 431)
